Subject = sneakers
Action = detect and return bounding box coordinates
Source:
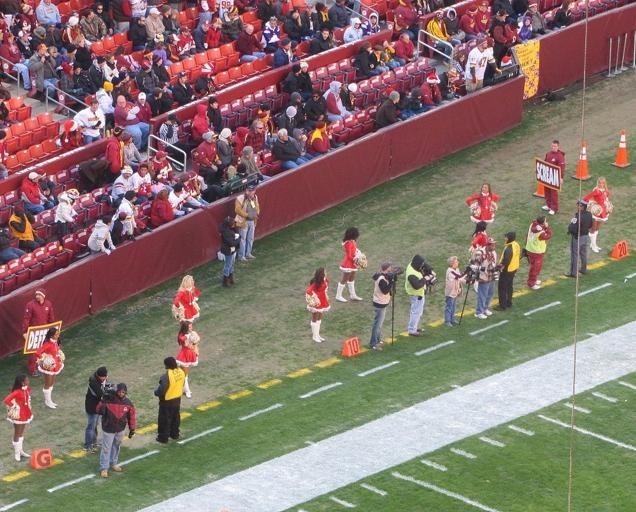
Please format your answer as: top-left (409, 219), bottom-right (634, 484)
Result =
top-left (246, 255), bottom-right (256, 260)
top-left (530, 280), bottom-right (541, 290)
top-left (445, 322), bottom-right (453, 328)
top-left (474, 313), bottom-right (488, 319)
top-left (237, 256), bottom-right (249, 263)
top-left (484, 309), bottom-right (493, 316)
top-left (408, 332), bottom-right (423, 337)
top-left (563, 272), bottom-right (575, 277)
top-left (541, 206), bottom-right (551, 211)
top-left (579, 269), bottom-right (586, 273)
top-left (371, 340), bottom-right (385, 351)
top-left (548, 210), bottom-right (555, 215)
top-left (418, 328), bottom-right (426, 332)
top-left (85, 444), bottom-right (122, 477)
top-left (452, 320), bottom-right (460, 325)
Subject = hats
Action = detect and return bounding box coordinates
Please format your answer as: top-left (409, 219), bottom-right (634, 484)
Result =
top-left (203, 92), bottom-right (301, 171)
top-left (35, 288), bottom-right (47, 299)
top-left (247, 182), bottom-right (256, 191)
top-left (465, 0), bottom-right (538, 45)
top-left (29, 127), bottom-right (197, 220)
top-left (97, 367), bottom-right (107, 377)
top-left (575, 199), bottom-right (589, 206)
top-left (390, 69), bottom-right (457, 100)
top-left (225, 217), bottom-right (235, 228)
top-left (19, 5), bottom-right (210, 106)
top-left (348, 83), bottom-right (358, 92)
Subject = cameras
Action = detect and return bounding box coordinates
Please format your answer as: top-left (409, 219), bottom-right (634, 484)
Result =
top-left (92, 120), bottom-right (102, 130)
top-left (387, 266), bottom-right (405, 284)
top-left (466, 264), bottom-right (486, 280)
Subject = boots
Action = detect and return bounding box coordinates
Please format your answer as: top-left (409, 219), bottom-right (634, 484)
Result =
top-left (336, 282), bottom-right (348, 302)
top-left (43, 386), bottom-right (58, 408)
top-left (223, 274), bottom-right (237, 289)
top-left (184, 375), bottom-right (191, 398)
top-left (589, 231), bottom-right (601, 253)
top-left (12, 437), bottom-right (31, 461)
top-left (311, 320), bottom-right (326, 342)
top-left (347, 281), bottom-right (363, 300)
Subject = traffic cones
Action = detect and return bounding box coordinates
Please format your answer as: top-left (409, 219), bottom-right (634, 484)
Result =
top-left (572, 142), bottom-right (593, 182)
top-left (532, 181), bottom-right (545, 198)
top-left (608, 128), bottom-right (632, 170)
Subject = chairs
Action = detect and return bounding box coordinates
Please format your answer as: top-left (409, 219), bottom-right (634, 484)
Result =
top-left (0, 0), bottom-right (636, 297)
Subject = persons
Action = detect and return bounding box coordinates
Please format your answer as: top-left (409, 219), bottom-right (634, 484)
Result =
top-left (366, 260), bottom-right (399, 351)
top-left (218, 216), bottom-right (241, 287)
top-left (81, 367), bottom-right (108, 453)
top-left (22, 287), bottom-right (55, 377)
top-left (1, 0), bottom-right (614, 260)
top-left (304, 267), bottom-right (332, 342)
top-left (335, 226), bottom-right (368, 303)
top-left (563, 197), bottom-right (592, 277)
top-left (153, 357), bottom-right (186, 443)
top-left (175, 320), bottom-right (200, 398)
top-left (443, 254), bottom-right (471, 328)
top-left (172, 274), bottom-right (201, 321)
top-left (405, 253), bottom-right (437, 335)
top-left (526, 215), bottom-right (554, 289)
top-left (471, 237), bottom-right (498, 319)
top-left (3, 374), bottom-right (35, 462)
top-left (492, 231), bottom-right (522, 312)
top-left (466, 221), bottom-right (489, 293)
top-left (95, 383), bottom-right (137, 478)
top-left (35, 327), bottom-right (66, 409)
